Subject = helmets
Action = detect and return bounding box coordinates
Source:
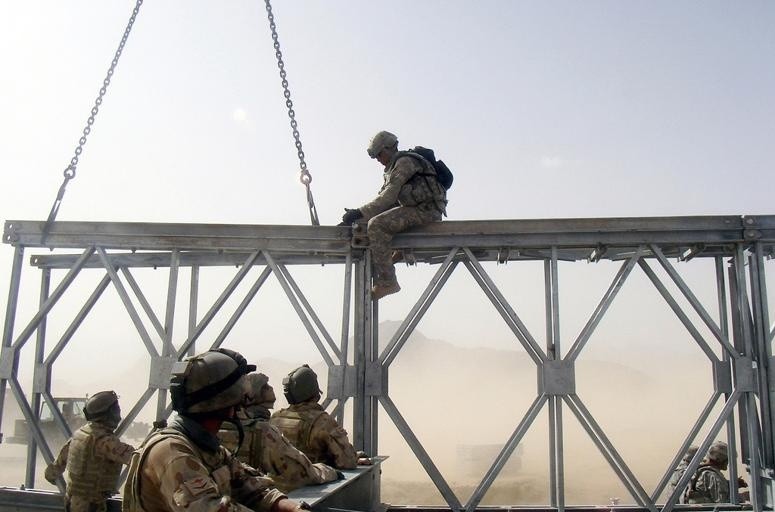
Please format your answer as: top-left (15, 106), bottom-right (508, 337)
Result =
top-left (681, 438), bottom-right (732, 465)
top-left (82, 391), bottom-right (117, 422)
top-left (170, 348), bottom-right (270, 415)
top-left (367, 131), bottom-right (398, 159)
top-left (282, 363), bottom-right (319, 404)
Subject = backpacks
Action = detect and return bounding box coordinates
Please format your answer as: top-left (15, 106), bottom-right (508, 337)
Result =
top-left (400, 146), bottom-right (454, 190)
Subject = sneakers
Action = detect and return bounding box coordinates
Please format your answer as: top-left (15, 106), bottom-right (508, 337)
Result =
top-left (371, 280), bottom-right (401, 302)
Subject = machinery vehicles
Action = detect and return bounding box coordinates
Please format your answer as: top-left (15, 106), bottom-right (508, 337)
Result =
top-left (5, 396), bottom-right (152, 461)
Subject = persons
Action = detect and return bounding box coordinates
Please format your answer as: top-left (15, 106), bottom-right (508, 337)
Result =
top-left (44, 390), bottom-right (138, 511)
top-left (122, 347), bottom-right (315, 512)
top-left (680, 440), bottom-right (749, 503)
top-left (218, 370), bottom-right (345, 494)
top-left (336, 130), bottom-right (448, 301)
top-left (269, 360), bottom-right (368, 470)
top-left (668, 441), bottom-right (707, 505)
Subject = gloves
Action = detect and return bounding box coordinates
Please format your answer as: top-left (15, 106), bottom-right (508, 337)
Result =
top-left (342, 207), bottom-right (363, 223)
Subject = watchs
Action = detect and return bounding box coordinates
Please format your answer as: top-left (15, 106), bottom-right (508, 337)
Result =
top-left (292, 499), bottom-right (310, 510)
